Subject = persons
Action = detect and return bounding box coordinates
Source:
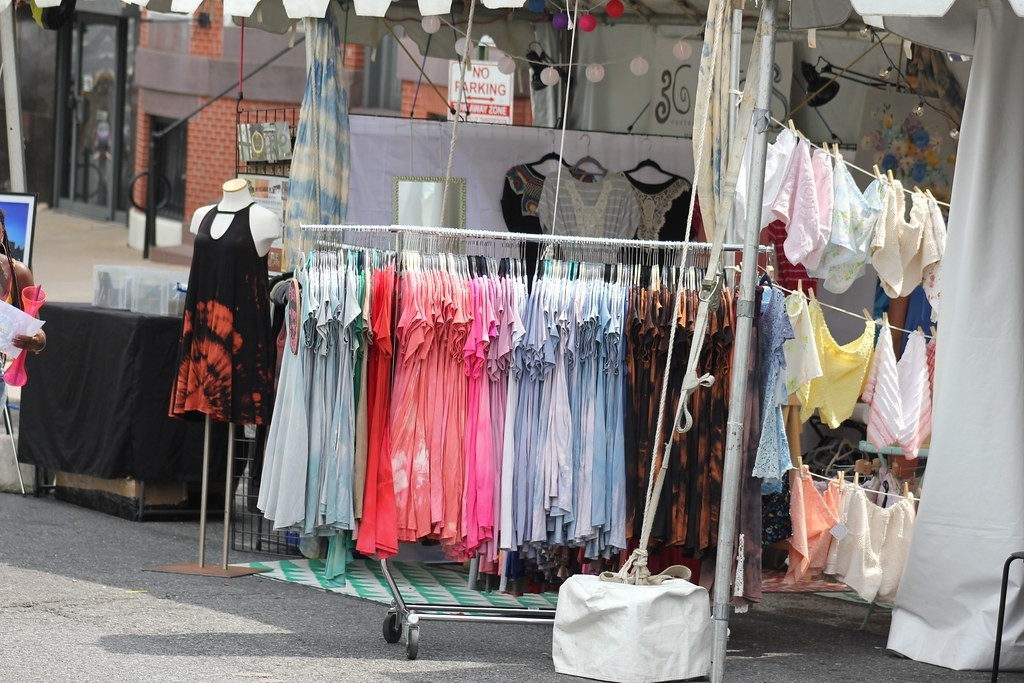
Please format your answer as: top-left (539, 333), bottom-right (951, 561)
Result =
top-left (0, 207), bottom-right (47, 417)
top-left (168, 177), bottom-right (282, 425)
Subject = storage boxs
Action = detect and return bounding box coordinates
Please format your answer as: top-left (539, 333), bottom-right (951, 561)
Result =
top-left (92, 264), bottom-right (190, 316)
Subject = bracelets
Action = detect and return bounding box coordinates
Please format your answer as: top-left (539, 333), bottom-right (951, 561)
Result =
top-left (34, 329), bottom-right (46, 355)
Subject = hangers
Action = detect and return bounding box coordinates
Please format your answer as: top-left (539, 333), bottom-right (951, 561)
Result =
top-left (299, 223), bottom-right (726, 294)
top-left (514, 126), bottom-right (697, 190)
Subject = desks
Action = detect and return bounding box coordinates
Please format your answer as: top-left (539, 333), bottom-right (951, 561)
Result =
top-left (18, 301), bottom-right (232, 519)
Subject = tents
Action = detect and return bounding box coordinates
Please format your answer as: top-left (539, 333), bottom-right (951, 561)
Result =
top-left (0, 0), bottom-right (1024, 683)
